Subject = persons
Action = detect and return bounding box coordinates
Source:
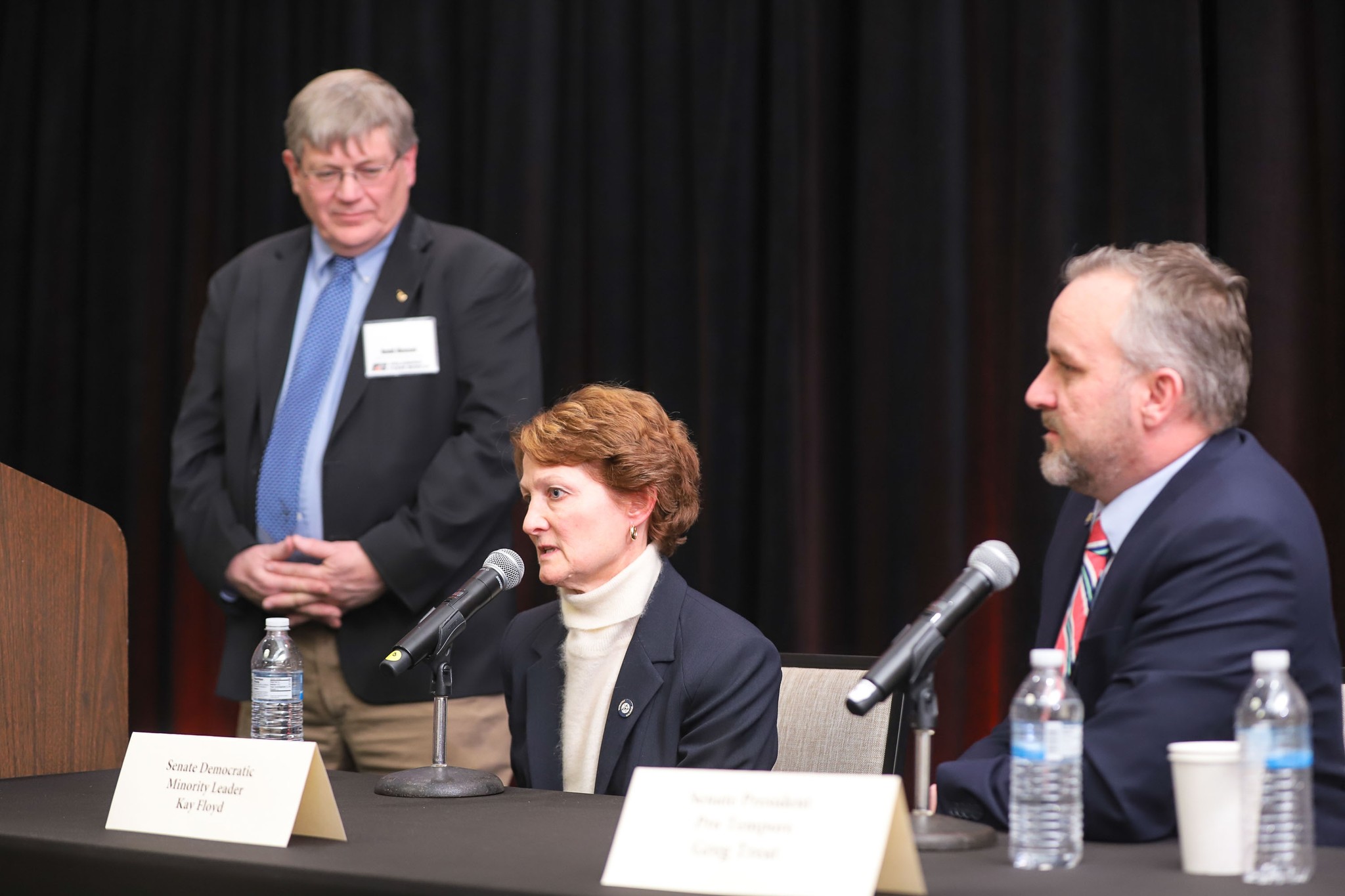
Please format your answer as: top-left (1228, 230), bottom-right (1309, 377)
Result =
top-left (498, 383), bottom-right (783, 795)
top-left (925, 241), bottom-right (1344, 844)
top-left (170, 69), bottom-right (545, 786)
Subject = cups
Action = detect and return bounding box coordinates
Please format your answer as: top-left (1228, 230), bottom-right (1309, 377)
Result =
top-left (1167, 740), bottom-right (1264, 876)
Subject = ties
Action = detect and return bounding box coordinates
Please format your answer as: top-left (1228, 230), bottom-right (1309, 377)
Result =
top-left (1041, 515), bottom-right (1110, 721)
top-left (256, 256), bottom-right (357, 549)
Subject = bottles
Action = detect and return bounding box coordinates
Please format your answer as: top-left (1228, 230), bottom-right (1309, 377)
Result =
top-left (1007, 647), bottom-right (1086, 869)
top-left (1232, 649), bottom-right (1316, 883)
top-left (251, 617), bottom-right (304, 741)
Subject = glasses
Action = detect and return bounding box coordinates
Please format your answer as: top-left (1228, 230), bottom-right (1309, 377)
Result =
top-left (294, 146), bottom-right (407, 187)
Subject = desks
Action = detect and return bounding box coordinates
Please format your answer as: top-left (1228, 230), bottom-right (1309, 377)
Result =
top-left (0, 765), bottom-right (1345, 896)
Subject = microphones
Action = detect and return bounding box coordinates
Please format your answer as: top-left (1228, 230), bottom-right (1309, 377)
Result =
top-left (846, 539), bottom-right (1021, 717)
top-left (379, 549), bottom-right (524, 681)
